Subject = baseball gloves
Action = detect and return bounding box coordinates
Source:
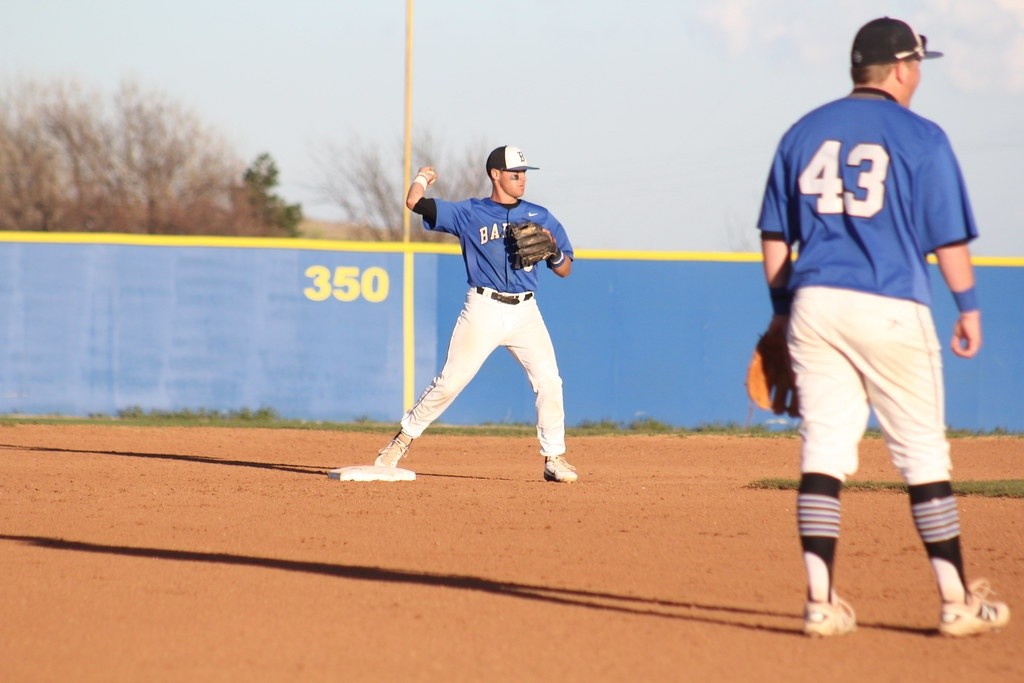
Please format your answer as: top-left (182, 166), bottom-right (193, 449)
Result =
top-left (746, 328), bottom-right (800, 418)
top-left (508, 219), bottom-right (560, 267)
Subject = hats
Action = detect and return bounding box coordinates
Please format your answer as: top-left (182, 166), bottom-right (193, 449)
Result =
top-left (850, 15), bottom-right (943, 67)
top-left (486, 144), bottom-right (540, 173)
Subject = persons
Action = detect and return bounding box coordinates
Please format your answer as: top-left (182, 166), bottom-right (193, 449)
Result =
top-left (372, 146), bottom-right (580, 483)
top-left (757, 19), bottom-right (1011, 638)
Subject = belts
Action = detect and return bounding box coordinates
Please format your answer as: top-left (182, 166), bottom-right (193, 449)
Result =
top-left (476, 287), bottom-right (533, 305)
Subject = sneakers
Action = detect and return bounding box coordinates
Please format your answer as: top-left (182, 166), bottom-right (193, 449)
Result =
top-left (937, 578), bottom-right (1010, 638)
top-left (375, 433), bottom-right (413, 468)
top-left (801, 589), bottom-right (858, 637)
top-left (543, 457), bottom-right (578, 483)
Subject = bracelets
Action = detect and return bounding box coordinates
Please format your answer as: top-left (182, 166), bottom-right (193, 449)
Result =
top-left (413, 175), bottom-right (427, 190)
top-left (416, 172), bottom-right (429, 182)
top-left (952, 287), bottom-right (977, 311)
